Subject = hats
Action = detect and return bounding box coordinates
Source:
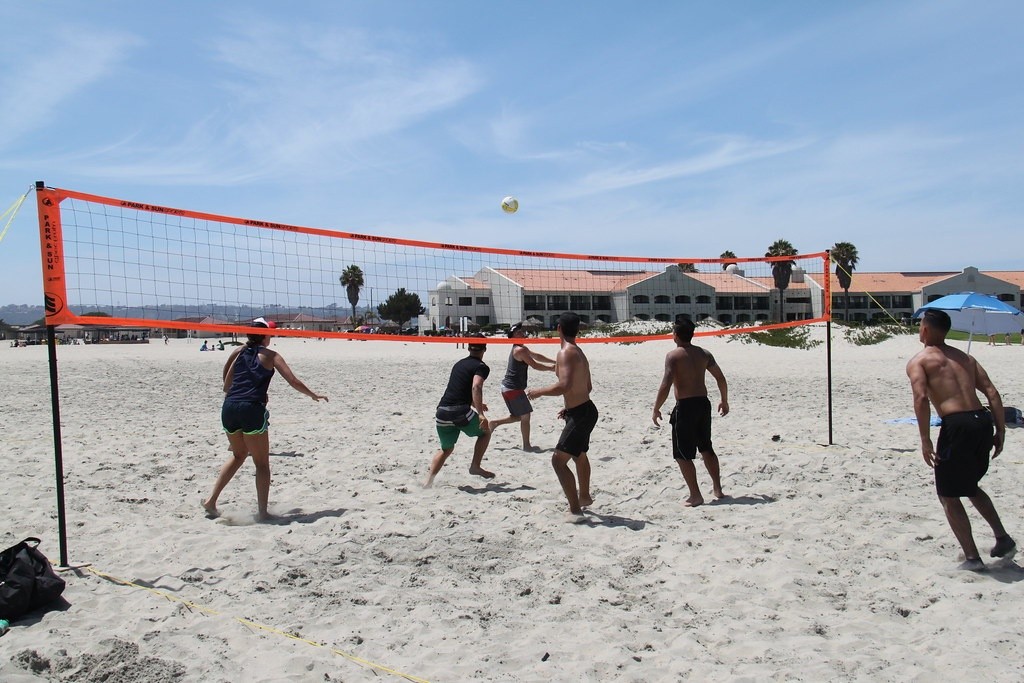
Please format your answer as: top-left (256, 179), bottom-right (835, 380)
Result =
top-left (469, 333), bottom-right (487, 351)
top-left (508, 321), bottom-right (522, 338)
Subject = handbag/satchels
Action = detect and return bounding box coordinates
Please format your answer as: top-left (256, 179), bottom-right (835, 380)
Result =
top-left (983, 404), bottom-right (1023, 425)
top-left (0, 537), bottom-right (66, 623)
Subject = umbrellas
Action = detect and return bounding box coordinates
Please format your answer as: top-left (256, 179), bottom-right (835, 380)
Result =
top-left (513, 317), bottom-right (543, 326)
top-left (914, 291), bottom-right (1024, 354)
top-left (355, 326), bottom-right (370, 330)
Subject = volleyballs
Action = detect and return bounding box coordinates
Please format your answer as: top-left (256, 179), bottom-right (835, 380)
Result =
top-left (501, 196), bottom-right (519, 213)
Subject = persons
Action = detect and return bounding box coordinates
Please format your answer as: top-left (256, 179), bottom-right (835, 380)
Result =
top-left (203, 317), bottom-right (329, 520)
top-left (365, 326), bottom-right (379, 334)
top-left (906, 308), bottom-right (1016, 571)
top-left (200, 340), bottom-right (208, 351)
top-left (218, 340), bottom-right (224, 351)
top-left (164, 335), bottom-right (168, 344)
top-left (488, 322), bottom-right (557, 452)
top-left (55, 332), bottom-right (148, 345)
top-left (988, 335), bottom-right (995, 345)
top-left (1021, 329), bottom-right (1024, 345)
top-left (526, 312), bottom-right (598, 524)
top-left (652, 314), bottom-right (729, 506)
top-left (10, 335), bottom-right (47, 347)
top-left (901, 316), bottom-right (907, 326)
top-left (423, 333), bottom-right (495, 489)
top-left (1005, 333), bottom-right (1011, 345)
top-left (210, 345), bottom-right (215, 351)
